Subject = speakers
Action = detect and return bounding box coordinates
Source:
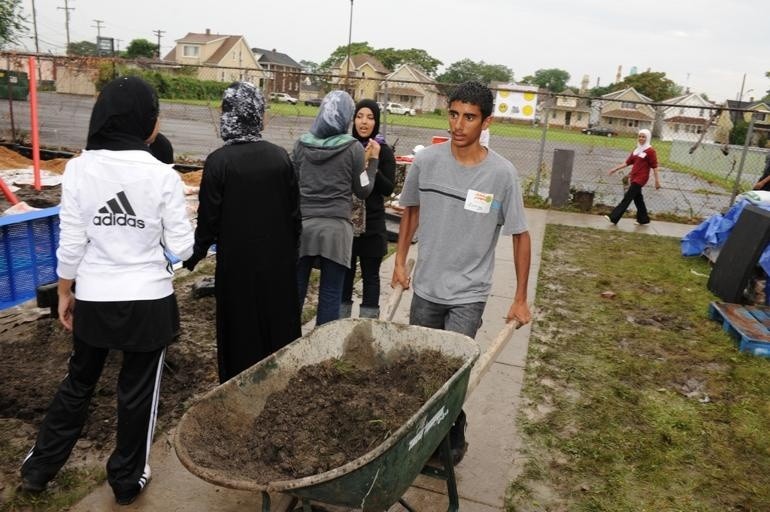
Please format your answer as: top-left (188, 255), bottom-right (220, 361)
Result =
top-left (339, 300), bottom-right (352, 320)
top-left (359, 303), bottom-right (380, 319)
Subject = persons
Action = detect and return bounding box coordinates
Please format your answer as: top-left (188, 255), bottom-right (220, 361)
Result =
top-left (183, 82), bottom-right (302, 383)
top-left (390, 80), bottom-right (531, 465)
top-left (604, 129), bottom-right (666, 228)
top-left (291, 89), bottom-right (381, 328)
top-left (18, 75), bottom-right (193, 504)
top-left (335, 100), bottom-right (397, 319)
top-left (752, 135), bottom-right (769, 191)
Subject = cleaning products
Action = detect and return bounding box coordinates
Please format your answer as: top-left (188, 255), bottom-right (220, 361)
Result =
top-left (172, 257), bottom-right (521, 512)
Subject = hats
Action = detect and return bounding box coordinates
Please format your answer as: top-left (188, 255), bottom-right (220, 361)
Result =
top-left (303, 97), bottom-right (324, 107)
top-left (580, 125), bottom-right (619, 138)
top-left (373, 102), bottom-right (384, 112)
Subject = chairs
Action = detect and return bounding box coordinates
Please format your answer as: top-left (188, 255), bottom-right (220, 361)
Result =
top-left (632, 220), bottom-right (650, 226)
top-left (119, 463), bottom-right (152, 505)
top-left (603, 214), bottom-right (615, 226)
top-left (440, 409), bottom-right (467, 464)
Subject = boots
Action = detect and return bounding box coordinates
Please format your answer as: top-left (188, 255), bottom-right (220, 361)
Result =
top-left (267, 91), bottom-right (298, 105)
top-left (384, 101), bottom-right (417, 116)
